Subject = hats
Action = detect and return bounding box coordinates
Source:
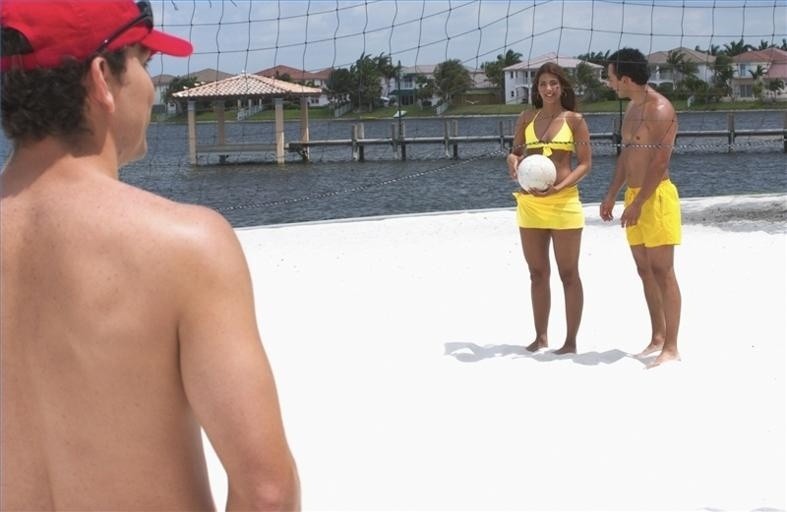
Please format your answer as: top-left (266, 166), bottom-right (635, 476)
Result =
top-left (0, 0), bottom-right (193, 71)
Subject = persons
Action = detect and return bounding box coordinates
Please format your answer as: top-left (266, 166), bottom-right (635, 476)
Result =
top-left (0, 0), bottom-right (303, 511)
top-left (599, 46), bottom-right (685, 368)
top-left (506, 62), bottom-right (592, 355)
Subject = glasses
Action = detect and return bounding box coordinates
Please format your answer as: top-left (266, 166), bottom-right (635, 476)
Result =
top-left (88, 0), bottom-right (152, 57)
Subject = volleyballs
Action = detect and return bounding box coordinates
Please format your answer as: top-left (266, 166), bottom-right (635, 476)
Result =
top-left (518, 153), bottom-right (557, 192)
